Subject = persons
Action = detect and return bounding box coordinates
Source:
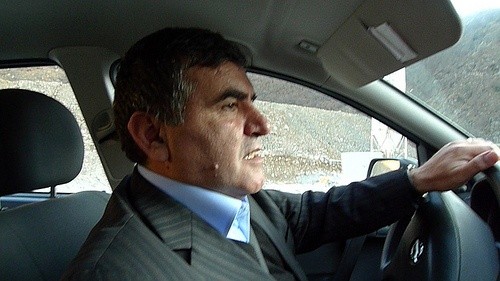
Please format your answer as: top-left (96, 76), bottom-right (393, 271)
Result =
top-left (60, 26), bottom-right (500, 281)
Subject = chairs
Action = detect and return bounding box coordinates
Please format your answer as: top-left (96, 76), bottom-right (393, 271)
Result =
top-left (0, 88), bottom-right (112, 281)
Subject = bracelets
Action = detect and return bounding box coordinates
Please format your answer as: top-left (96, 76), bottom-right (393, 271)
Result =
top-left (408, 164), bottom-right (416, 172)
